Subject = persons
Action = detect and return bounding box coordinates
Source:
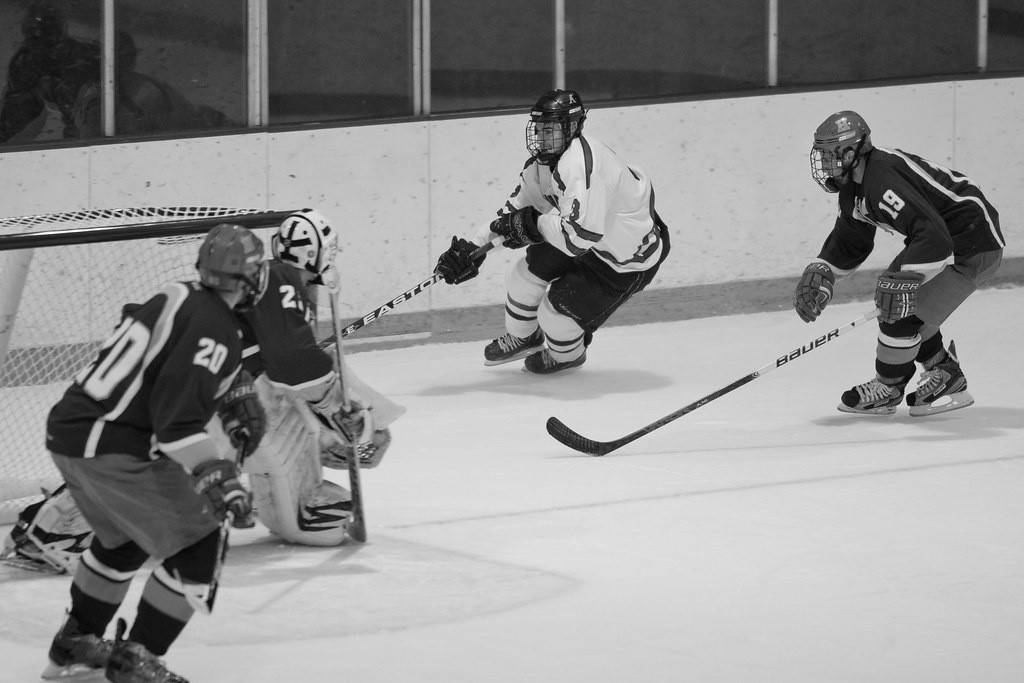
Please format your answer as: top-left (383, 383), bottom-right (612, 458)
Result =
top-left (435, 89), bottom-right (671, 373)
top-left (0, 6), bottom-right (234, 145)
top-left (44, 223), bottom-right (269, 683)
top-left (0, 209), bottom-right (390, 576)
top-left (793, 110), bottom-right (1006, 414)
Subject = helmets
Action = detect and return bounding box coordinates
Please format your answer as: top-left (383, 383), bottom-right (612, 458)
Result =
top-left (195, 224), bottom-right (268, 308)
top-left (810, 110), bottom-right (873, 194)
top-left (271, 207), bottom-right (339, 275)
top-left (525, 87), bottom-right (588, 168)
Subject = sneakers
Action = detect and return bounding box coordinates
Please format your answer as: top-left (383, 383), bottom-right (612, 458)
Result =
top-left (837, 378), bottom-right (905, 415)
top-left (40, 617), bottom-right (115, 681)
top-left (520, 344), bottom-right (588, 374)
top-left (906, 357), bottom-right (975, 417)
top-left (100, 636), bottom-right (192, 683)
top-left (483, 322), bottom-right (548, 366)
top-left (0, 492), bottom-right (80, 576)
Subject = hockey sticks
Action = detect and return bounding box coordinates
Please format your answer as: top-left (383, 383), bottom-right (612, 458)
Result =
top-left (172, 426), bottom-right (252, 619)
top-left (544, 308), bottom-right (884, 458)
top-left (328, 292), bottom-right (368, 544)
top-left (316, 233), bottom-right (510, 350)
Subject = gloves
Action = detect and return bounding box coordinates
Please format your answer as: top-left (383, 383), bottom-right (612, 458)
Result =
top-left (309, 378), bottom-right (374, 452)
top-left (433, 235), bottom-right (487, 285)
top-left (220, 372), bottom-right (267, 460)
top-left (490, 205), bottom-right (545, 250)
top-left (189, 459), bottom-right (256, 529)
top-left (793, 262), bottom-right (836, 323)
top-left (874, 269), bottom-right (926, 325)
